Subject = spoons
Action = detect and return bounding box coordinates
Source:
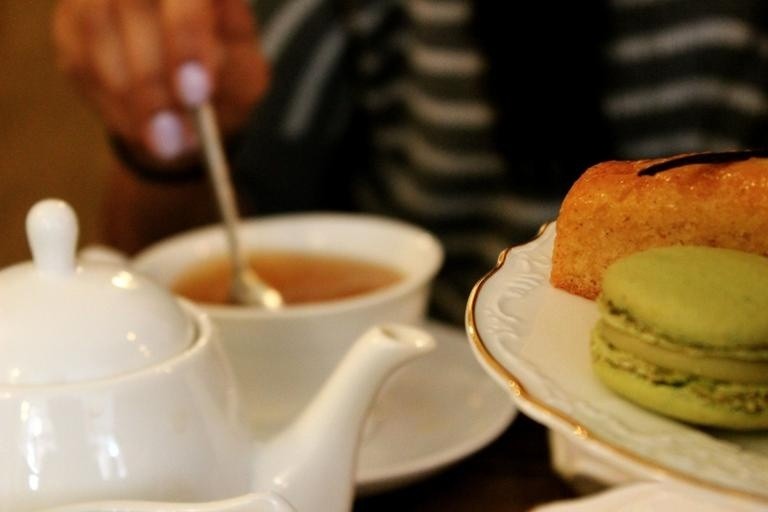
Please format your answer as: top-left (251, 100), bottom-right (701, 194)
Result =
top-left (195, 97), bottom-right (283, 307)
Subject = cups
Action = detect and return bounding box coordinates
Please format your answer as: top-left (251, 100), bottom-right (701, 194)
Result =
top-left (138, 209), bottom-right (442, 426)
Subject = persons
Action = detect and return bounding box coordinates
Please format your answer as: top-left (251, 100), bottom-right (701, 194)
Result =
top-left (47, 0), bottom-right (767, 496)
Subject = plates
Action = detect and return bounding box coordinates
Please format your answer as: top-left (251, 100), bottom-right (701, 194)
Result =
top-left (466, 219), bottom-right (767, 504)
top-left (352, 314), bottom-right (516, 485)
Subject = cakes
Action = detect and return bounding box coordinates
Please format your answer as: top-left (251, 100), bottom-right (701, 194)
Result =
top-left (549, 149), bottom-right (768, 299)
top-left (588, 245), bottom-right (767, 432)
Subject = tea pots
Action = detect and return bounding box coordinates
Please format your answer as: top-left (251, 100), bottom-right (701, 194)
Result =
top-left (1, 196), bottom-right (435, 512)
